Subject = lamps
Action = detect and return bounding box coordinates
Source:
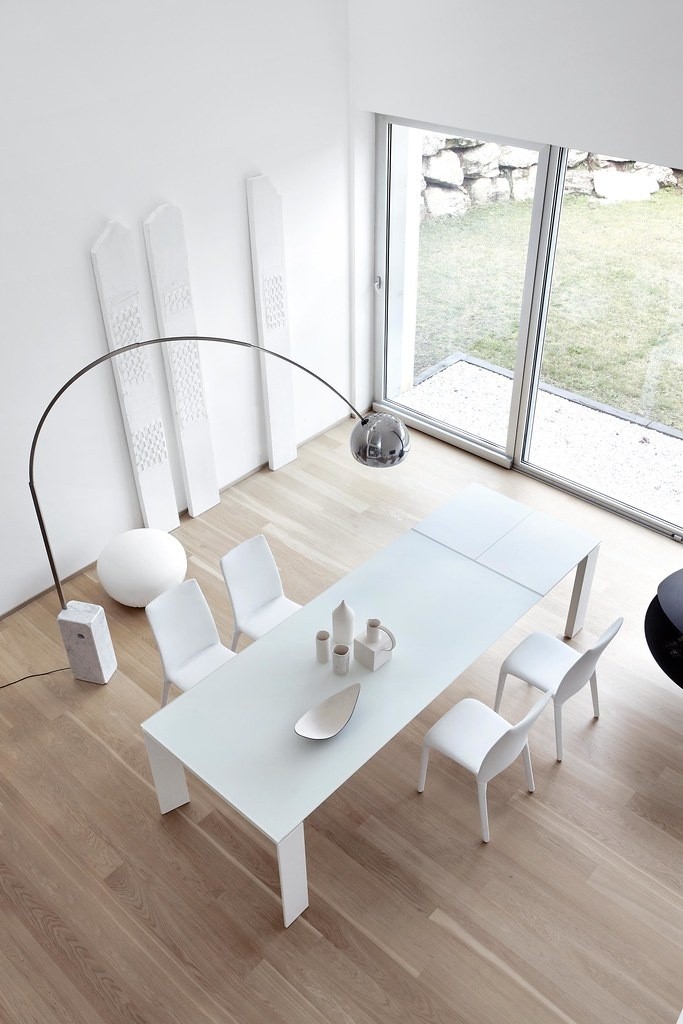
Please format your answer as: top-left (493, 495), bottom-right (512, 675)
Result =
top-left (28, 335), bottom-right (411, 685)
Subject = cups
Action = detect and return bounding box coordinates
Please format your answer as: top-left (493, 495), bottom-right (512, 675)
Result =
top-left (332, 644), bottom-right (350, 677)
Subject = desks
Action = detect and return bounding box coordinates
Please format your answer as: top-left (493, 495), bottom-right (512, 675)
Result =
top-left (141, 479), bottom-right (603, 926)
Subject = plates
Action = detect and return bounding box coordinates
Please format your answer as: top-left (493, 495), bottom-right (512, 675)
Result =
top-left (294, 682), bottom-right (362, 740)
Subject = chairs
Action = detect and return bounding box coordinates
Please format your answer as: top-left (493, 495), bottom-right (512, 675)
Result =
top-left (219, 534), bottom-right (303, 652)
top-left (145, 578), bottom-right (237, 709)
top-left (417, 687), bottom-right (557, 847)
top-left (495, 618), bottom-right (624, 762)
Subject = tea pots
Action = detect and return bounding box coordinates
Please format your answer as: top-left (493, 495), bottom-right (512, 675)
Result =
top-left (353, 618), bottom-right (396, 672)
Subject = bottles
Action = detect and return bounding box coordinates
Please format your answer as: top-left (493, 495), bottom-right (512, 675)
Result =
top-left (316, 631), bottom-right (331, 664)
